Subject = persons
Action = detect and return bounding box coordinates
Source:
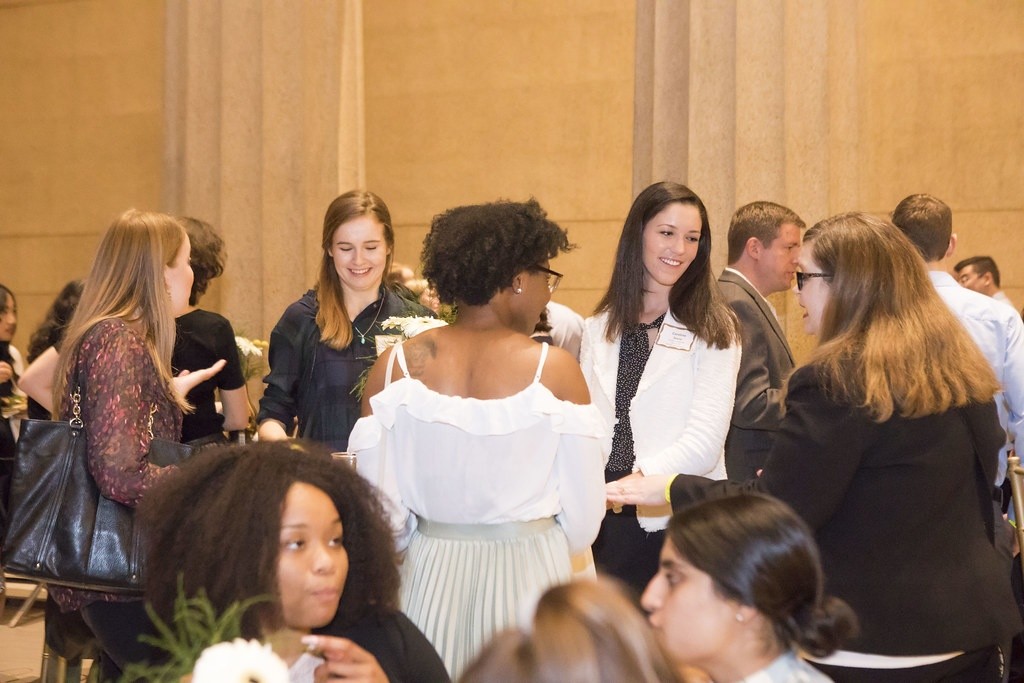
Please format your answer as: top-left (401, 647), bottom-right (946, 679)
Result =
top-left (606, 194), bottom-right (1024, 683)
top-left (0, 280), bottom-right (87, 568)
top-left (531, 290), bottom-right (584, 365)
top-left (344, 200), bottom-right (608, 683)
top-left (644, 492), bottom-right (859, 683)
top-left (381, 256), bottom-right (441, 318)
top-left (258, 190), bottom-right (446, 451)
top-left (581, 181), bottom-right (742, 603)
top-left (144, 441), bottom-right (451, 683)
top-left (168, 215), bottom-right (252, 451)
top-left (54, 208), bottom-right (230, 683)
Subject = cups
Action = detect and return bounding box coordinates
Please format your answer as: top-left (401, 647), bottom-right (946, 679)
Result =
top-left (331, 453), bottom-right (356, 470)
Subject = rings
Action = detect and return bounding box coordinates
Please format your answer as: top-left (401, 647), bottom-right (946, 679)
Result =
top-left (612, 501), bottom-right (624, 514)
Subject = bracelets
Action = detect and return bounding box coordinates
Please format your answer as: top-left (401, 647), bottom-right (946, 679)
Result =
top-left (665, 483), bottom-right (671, 506)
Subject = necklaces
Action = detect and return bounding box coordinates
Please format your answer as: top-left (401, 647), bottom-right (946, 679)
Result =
top-left (355, 287), bottom-right (386, 345)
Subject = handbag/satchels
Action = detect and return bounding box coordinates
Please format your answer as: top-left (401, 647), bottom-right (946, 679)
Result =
top-left (1, 329), bottom-right (205, 595)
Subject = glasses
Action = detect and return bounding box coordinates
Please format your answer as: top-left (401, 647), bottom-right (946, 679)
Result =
top-left (796, 272), bottom-right (832, 291)
top-left (533, 264), bottom-right (564, 294)
